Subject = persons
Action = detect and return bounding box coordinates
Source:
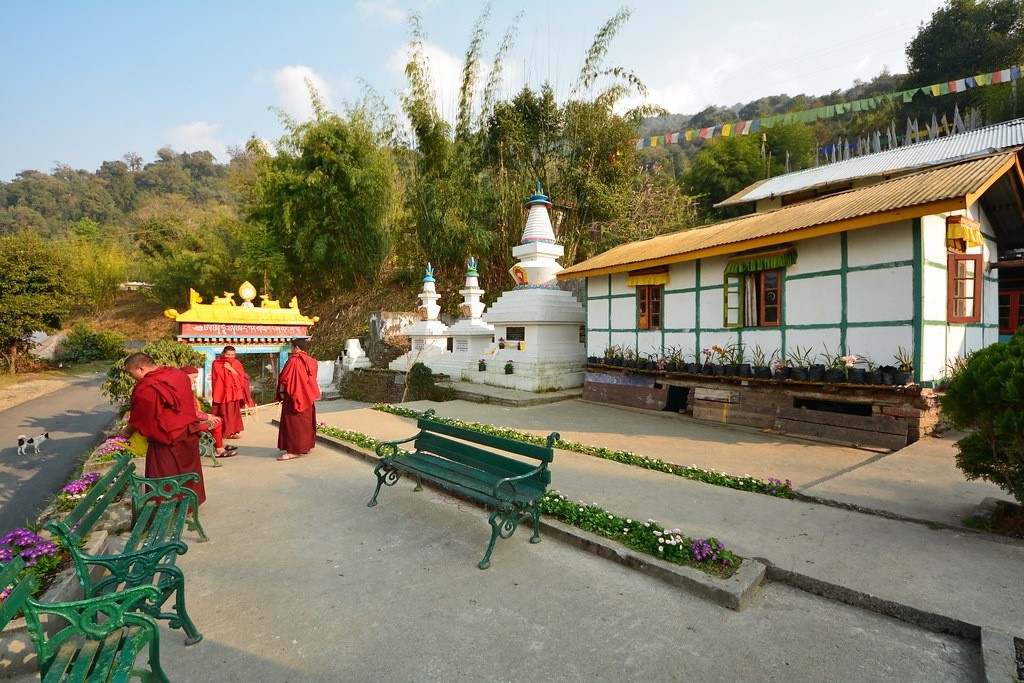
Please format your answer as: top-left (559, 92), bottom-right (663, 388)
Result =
top-left (275, 338), bottom-right (320, 460)
top-left (118, 353), bottom-right (206, 525)
top-left (181, 365), bottom-right (238, 458)
top-left (212, 346), bottom-right (255, 439)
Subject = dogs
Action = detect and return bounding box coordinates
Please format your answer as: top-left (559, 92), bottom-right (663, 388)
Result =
top-left (16, 432), bottom-right (49, 456)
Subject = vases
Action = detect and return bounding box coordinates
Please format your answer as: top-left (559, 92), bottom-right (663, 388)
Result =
top-left (517, 346), bottom-right (521, 350)
top-left (505, 369), bottom-right (513, 374)
top-left (586, 356), bottom-right (915, 381)
top-left (500, 345), bottom-right (504, 349)
top-left (479, 365), bottom-right (486, 371)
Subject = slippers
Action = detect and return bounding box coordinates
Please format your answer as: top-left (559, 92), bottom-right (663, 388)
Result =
top-left (214, 450), bottom-right (237, 457)
top-left (225, 444), bottom-right (237, 450)
top-left (277, 453), bottom-right (298, 460)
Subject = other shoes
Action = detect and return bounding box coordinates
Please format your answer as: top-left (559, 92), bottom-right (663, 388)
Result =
top-left (228, 434), bottom-right (241, 439)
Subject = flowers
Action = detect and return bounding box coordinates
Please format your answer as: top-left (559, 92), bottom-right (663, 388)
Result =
top-left (652, 357), bottom-right (668, 369)
top-left (686, 348), bottom-right (712, 363)
top-left (478, 358), bottom-right (488, 366)
top-left (711, 344), bottom-right (747, 364)
top-left (503, 360), bottom-right (514, 369)
top-left (836, 353), bottom-right (856, 379)
top-left (498, 337), bottom-right (504, 345)
top-left (0, 518), bottom-right (86, 603)
top-left (93, 436), bottom-right (134, 461)
top-left (518, 342), bottom-right (520, 346)
top-left (770, 356), bottom-right (790, 375)
top-left (59, 469), bottom-right (117, 511)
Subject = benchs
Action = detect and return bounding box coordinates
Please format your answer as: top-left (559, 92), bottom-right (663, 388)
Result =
top-left (197, 420), bottom-right (223, 467)
top-left (41, 451), bottom-right (210, 646)
top-left (366, 407), bottom-right (561, 570)
top-left (0, 555), bottom-right (172, 683)
